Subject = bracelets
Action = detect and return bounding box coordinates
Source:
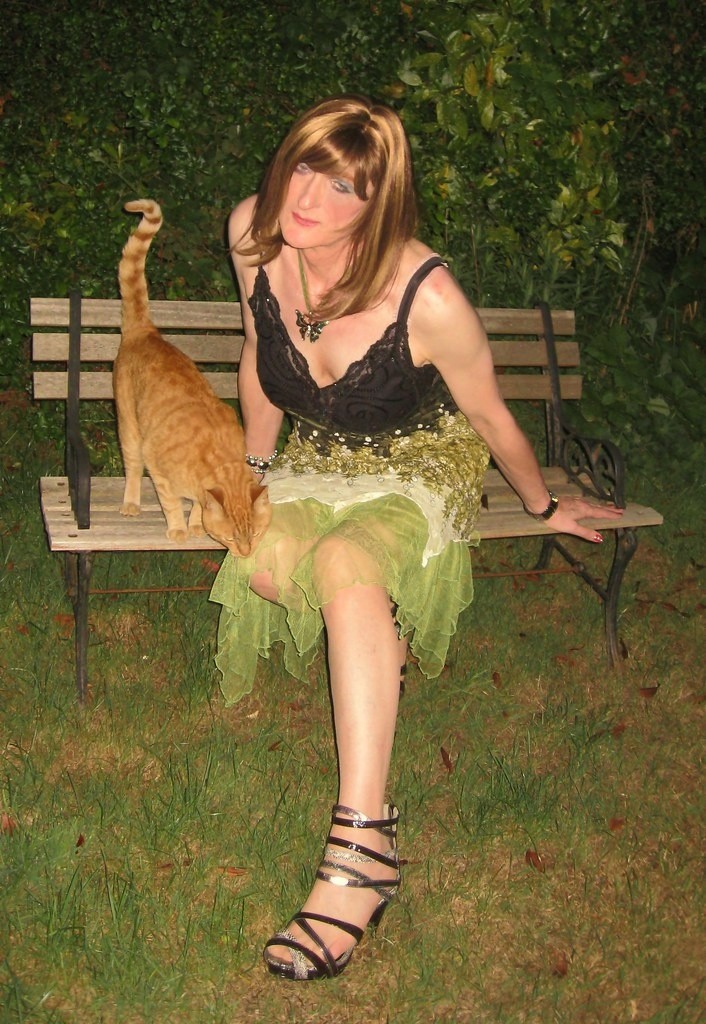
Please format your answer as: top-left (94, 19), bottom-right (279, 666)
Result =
top-left (244, 450), bottom-right (279, 473)
top-left (523, 490), bottom-right (557, 522)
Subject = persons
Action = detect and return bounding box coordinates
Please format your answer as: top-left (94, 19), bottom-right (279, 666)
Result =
top-left (209, 96), bottom-right (622, 979)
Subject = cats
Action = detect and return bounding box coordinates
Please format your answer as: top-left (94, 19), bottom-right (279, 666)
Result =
top-left (113, 199), bottom-right (272, 558)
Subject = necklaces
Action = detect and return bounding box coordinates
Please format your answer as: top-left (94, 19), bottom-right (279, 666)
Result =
top-left (295, 247), bottom-right (329, 343)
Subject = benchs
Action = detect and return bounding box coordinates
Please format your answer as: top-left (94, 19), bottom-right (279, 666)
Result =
top-left (30, 296), bottom-right (665, 703)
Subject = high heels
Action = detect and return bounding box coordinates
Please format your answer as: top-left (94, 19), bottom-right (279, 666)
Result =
top-left (263, 804), bottom-right (401, 981)
